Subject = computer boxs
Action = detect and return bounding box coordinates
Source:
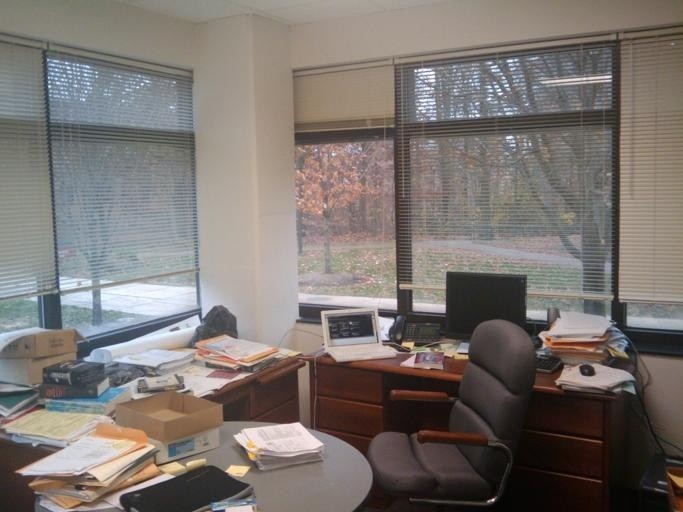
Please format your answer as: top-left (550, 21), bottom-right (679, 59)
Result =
top-left (638, 455), bottom-right (683, 512)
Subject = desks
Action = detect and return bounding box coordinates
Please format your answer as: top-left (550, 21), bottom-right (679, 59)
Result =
top-left (0, 341), bottom-right (306, 512)
top-left (33, 420), bottom-right (374, 511)
top-left (307, 327), bottom-right (635, 511)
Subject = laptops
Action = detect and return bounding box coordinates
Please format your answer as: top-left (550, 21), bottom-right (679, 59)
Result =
top-left (320, 306), bottom-right (397, 363)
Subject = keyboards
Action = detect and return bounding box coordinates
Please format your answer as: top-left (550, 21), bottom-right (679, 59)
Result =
top-left (535, 352), bottom-right (562, 374)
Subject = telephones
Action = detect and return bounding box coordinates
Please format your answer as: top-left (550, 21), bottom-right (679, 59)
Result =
top-left (389, 315), bottom-right (444, 347)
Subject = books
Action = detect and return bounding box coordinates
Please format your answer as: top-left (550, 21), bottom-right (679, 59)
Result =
top-left (414, 351), bottom-right (444, 369)
top-left (38, 359), bottom-right (193, 416)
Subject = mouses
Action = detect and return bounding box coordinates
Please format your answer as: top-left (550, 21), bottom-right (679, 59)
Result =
top-left (579, 364), bottom-right (595, 376)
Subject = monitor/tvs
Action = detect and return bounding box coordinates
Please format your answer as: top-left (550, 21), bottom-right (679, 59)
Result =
top-left (445, 271), bottom-right (527, 355)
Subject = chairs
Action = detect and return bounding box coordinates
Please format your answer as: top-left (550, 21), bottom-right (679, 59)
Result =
top-left (366, 318), bottom-right (537, 511)
